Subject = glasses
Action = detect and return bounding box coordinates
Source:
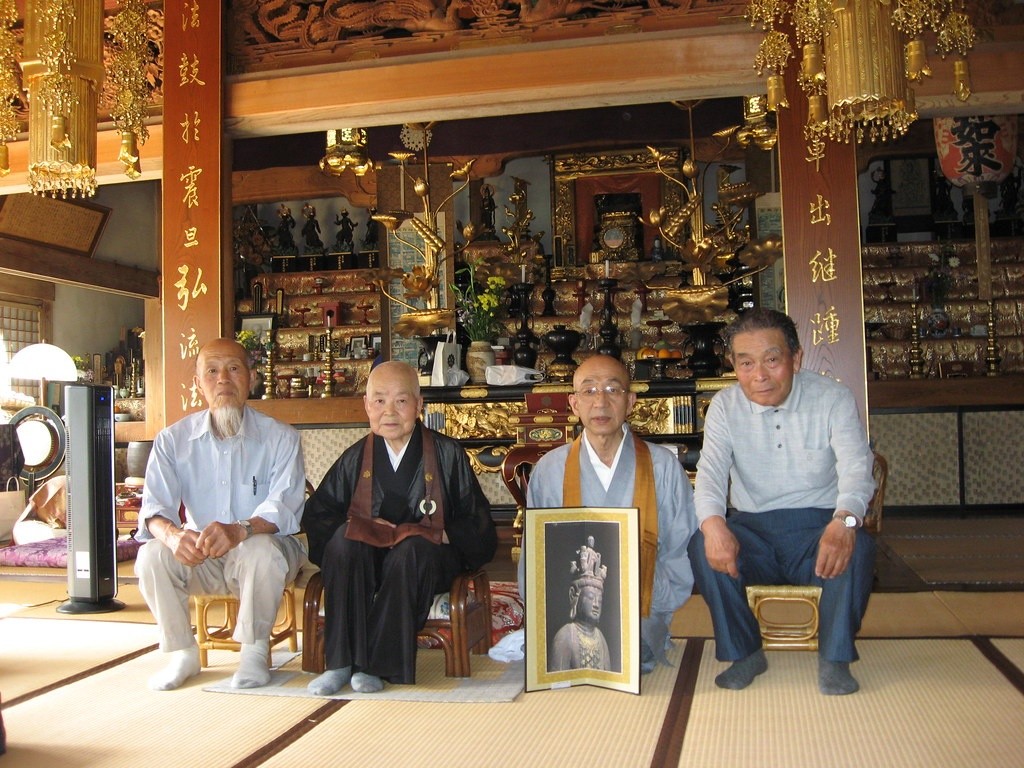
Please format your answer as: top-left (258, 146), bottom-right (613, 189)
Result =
top-left (570, 386), bottom-right (628, 399)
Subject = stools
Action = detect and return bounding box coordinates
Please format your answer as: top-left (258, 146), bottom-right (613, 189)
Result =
top-left (302, 560), bottom-right (491, 679)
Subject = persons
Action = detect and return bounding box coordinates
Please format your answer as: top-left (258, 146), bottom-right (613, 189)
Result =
top-left (134, 337), bottom-right (304, 692)
top-left (365, 206), bottom-right (379, 244)
top-left (301, 214), bottom-right (325, 246)
top-left (278, 215), bottom-right (296, 250)
top-left (868, 172), bottom-right (1019, 216)
top-left (651, 234), bottom-right (664, 265)
top-left (549, 534), bottom-right (611, 674)
top-left (517, 355), bottom-right (699, 675)
top-left (687, 305), bottom-right (877, 696)
top-left (334, 209), bottom-right (358, 247)
top-left (301, 362), bottom-right (497, 696)
top-left (480, 187), bottom-right (495, 229)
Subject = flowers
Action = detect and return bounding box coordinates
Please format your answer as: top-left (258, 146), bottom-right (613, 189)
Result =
top-left (448, 250), bottom-right (520, 343)
top-left (928, 237), bottom-right (972, 309)
top-left (233, 329), bottom-right (275, 367)
top-left (72, 351), bottom-right (94, 385)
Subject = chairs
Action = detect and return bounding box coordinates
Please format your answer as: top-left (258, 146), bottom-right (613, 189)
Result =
top-left (192, 472), bottom-right (316, 669)
top-left (699, 448), bottom-right (889, 649)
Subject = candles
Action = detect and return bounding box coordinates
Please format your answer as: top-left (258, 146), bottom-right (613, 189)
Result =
top-left (913, 288), bottom-right (916, 300)
top-left (327, 316), bottom-right (330, 327)
top-left (522, 265), bottom-right (525, 283)
top-left (605, 260), bottom-right (609, 278)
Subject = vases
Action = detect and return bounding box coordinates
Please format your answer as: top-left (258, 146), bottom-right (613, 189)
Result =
top-left (466, 341), bottom-right (496, 382)
top-left (250, 369), bottom-right (263, 396)
top-left (928, 308), bottom-right (950, 338)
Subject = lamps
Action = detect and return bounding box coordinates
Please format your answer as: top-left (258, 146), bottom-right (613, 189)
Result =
top-left (7, 338), bottom-right (76, 421)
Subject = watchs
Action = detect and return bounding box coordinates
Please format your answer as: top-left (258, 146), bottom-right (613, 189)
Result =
top-left (237, 519), bottom-right (252, 539)
top-left (834, 514), bottom-right (859, 531)
